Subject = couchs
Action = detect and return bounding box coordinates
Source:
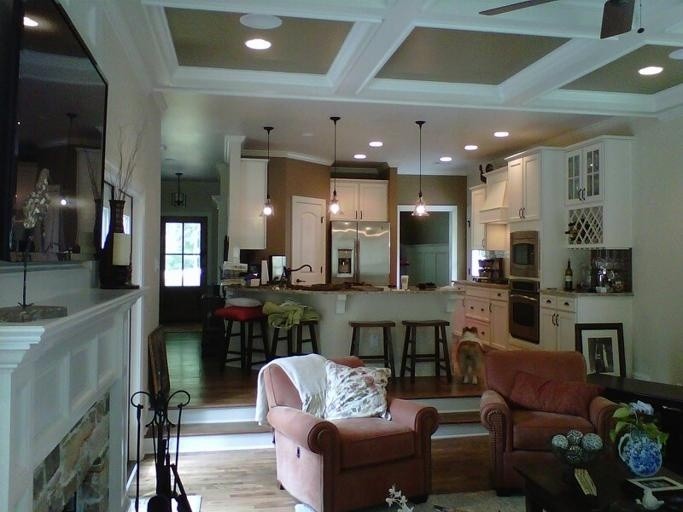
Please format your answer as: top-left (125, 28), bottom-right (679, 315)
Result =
top-left (479, 347), bottom-right (628, 495)
top-left (262, 355), bottom-right (440, 510)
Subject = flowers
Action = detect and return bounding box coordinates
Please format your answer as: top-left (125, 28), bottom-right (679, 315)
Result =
top-left (609, 400), bottom-right (668, 454)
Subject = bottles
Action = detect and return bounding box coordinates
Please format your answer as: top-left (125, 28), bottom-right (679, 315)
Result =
top-left (565, 260), bottom-right (573, 290)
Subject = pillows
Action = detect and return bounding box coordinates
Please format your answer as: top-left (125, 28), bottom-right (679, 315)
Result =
top-left (224, 298), bottom-right (262, 308)
top-left (324, 359), bottom-right (393, 421)
top-left (508, 371), bottom-right (604, 415)
top-left (214, 308), bottom-right (265, 320)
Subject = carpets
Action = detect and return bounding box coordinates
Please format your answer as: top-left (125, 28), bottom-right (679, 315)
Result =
top-left (293, 490), bottom-right (530, 512)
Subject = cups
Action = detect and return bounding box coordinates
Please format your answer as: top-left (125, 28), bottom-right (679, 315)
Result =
top-left (401, 275), bottom-right (408, 289)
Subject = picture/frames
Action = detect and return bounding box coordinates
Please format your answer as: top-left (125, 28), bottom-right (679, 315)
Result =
top-left (574, 323), bottom-right (627, 377)
top-left (626, 477), bottom-right (682, 492)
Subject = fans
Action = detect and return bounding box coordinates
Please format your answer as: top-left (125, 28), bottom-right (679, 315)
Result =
top-left (478, 0), bottom-right (645, 39)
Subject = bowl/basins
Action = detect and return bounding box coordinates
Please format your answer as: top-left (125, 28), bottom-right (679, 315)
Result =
top-left (548, 432), bottom-right (609, 468)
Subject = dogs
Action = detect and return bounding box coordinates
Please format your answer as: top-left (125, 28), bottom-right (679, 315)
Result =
top-left (457, 326), bottom-right (483, 385)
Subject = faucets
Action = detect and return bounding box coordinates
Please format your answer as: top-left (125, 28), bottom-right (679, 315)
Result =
top-left (287, 264), bottom-right (312, 287)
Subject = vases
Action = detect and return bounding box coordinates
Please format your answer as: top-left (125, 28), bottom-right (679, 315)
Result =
top-left (627, 435), bottom-right (663, 476)
top-left (97, 199), bottom-right (133, 288)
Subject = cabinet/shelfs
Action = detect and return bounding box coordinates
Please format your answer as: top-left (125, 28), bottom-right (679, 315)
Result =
top-left (451, 279), bottom-right (509, 353)
top-left (504, 145), bottom-right (566, 234)
top-left (468, 185), bottom-right (506, 284)
top-left (328, 179), bottom-right (389, 223)
top-left (540, 290), bottom-right (634, 377)
top-left (560, 135), bottom-right (635, 252)
top-left (289, 195), bottom-right (326, 286)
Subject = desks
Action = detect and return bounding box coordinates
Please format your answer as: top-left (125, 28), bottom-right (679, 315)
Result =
top-left (584, 374), bottom-right (683, 472)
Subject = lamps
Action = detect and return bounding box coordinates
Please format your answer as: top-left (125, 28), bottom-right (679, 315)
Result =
top-left (411, 120), bottom-right (430, 216)
top-left (327, 116), bottom-right (344, 215)
top-left (258, 127), bottom-right (276, 217)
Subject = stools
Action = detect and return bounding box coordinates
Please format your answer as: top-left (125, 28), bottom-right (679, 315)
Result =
top-left (220, 315), bottom-right (272, 378)
top-left (400, 319), bottom-right (453, 384)
top-left (349, 320), bottom-right (395, 384)
top-left (270, 319), bottom-right (319, 359)
